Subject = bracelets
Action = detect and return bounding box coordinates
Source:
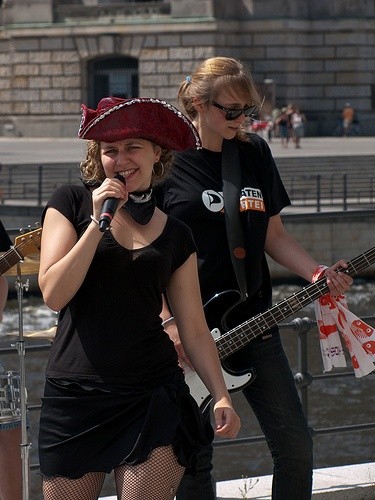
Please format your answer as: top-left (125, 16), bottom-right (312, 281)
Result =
top-left (160, 316), bottom-right (175, 326)
top-left (89, 214), bottom-right (111, 230)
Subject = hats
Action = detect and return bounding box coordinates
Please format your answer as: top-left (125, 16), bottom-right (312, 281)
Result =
top-left (78, 97), bottom-right (202, 151)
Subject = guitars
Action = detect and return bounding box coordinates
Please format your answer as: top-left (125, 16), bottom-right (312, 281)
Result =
top-left (0, 222), bottom-right (44, 278)
top-left (161, 245), bottom-right (375, 414)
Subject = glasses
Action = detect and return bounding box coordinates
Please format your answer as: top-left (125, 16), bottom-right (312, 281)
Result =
top-left (210, 101), bottom-right (257, 120)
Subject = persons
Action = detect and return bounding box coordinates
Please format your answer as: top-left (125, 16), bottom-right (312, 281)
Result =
top-left (158, 56), bottom-right (357, 500)
top-left (0, 220), bottom-right (15, 324)
top-left (38, 96), bottom-right (241, 499)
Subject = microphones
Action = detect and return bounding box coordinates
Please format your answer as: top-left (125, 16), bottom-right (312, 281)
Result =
top-left (99, 174), bottom-right (126, 232)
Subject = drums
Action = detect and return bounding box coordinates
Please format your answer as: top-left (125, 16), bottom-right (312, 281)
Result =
top-left (0, 414), bottom-right (29, 500)
